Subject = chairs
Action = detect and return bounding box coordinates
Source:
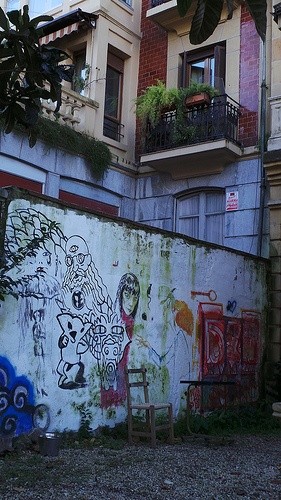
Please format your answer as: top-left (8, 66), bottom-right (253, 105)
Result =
top-left (122, 365), bottom-right (175, 446)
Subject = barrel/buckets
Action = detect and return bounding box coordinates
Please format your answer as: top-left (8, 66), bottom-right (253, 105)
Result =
top-left (39, 432), bottom-right (61, 455)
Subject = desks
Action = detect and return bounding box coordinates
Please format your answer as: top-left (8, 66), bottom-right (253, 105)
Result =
top-left (180, 378), bottom-right (236, 429)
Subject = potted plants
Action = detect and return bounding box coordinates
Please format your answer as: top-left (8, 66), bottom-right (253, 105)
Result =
top-left (181, 85), bottom-right (214, 106)
